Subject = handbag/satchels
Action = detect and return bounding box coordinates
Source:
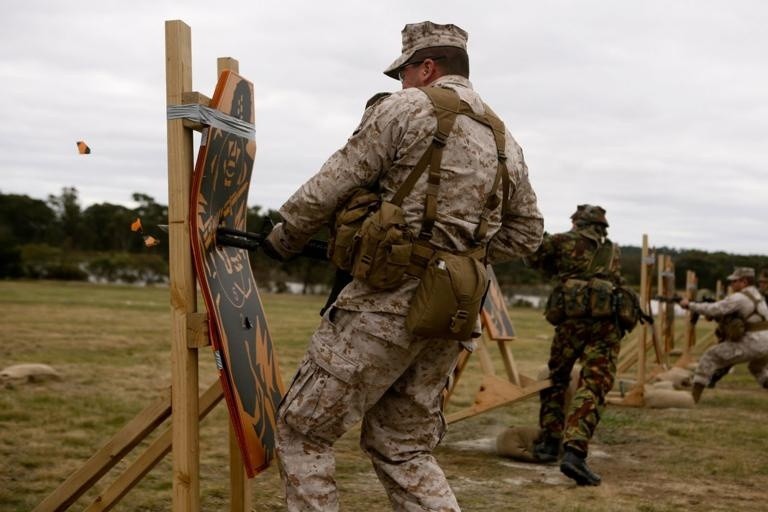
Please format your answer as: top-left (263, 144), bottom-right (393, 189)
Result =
top-left (407, 252), bottom-right (489, 341)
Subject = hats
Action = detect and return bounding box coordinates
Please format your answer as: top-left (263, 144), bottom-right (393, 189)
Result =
top-left (383, 21), bottom-right (469, 81)
top-left (725, 266), bottom-right (755, 281)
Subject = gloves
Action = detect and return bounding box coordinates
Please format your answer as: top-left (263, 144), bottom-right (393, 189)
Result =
top-left (263, 222), bottom-right (304, 264)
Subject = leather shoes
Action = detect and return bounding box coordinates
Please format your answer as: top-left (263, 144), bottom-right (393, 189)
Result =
top-left (534, 432), bottom-right (561, 462)
top-left (560, 449), bottom-right (601, 485)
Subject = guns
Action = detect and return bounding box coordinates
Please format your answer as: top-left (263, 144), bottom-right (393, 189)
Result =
top-left (139, 217), bottom-right (353, 321)
top-left (650, 294), bottom-right (719, 325)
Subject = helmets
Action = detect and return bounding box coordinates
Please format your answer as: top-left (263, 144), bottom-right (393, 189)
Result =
top-left (569, 204), bottom-right (608, 227)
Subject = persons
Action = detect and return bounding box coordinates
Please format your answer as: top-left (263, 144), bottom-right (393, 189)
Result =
top-left (261, 20), bottom-right (545, 512)
top-left (675, 266), bottom-right (768, 407)
top-left (519, 205), bottom-right (650, 486)
top-left (707, 267), bottom-right (768, 388)
